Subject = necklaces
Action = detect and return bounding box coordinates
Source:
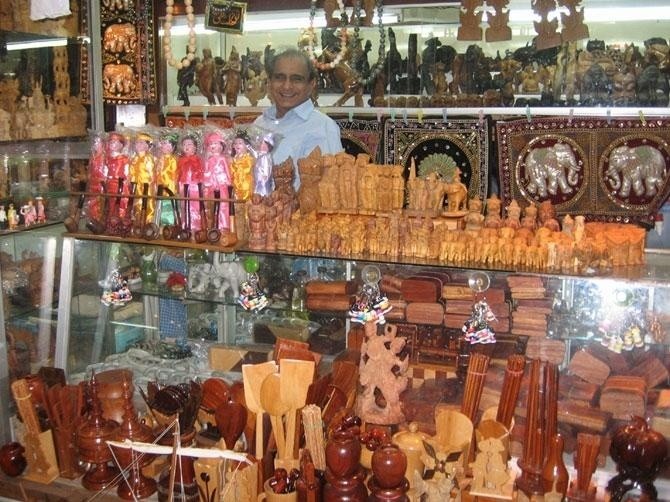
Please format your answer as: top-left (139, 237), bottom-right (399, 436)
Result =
top-left (207, 1), bottom-right (234, 11)
top-left (162, 0), bottom-right (197, 71)
top-left (306, 1), bottom-right (348, 70)
top-left (349, 1), bottom-right (386, 86)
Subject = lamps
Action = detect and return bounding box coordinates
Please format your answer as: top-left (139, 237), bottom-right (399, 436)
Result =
top-left (2, 0), bottom-right (88, 54)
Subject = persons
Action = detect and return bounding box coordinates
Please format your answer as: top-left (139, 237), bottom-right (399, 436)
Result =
top-left (197, 48), bottom-right (218, 106)
top-left (221, 45), bottom-right (241, 108)
top-left (238, 47), bottom-right (263, 107)
top-left (0, 199), bottom-right (48, 234)
top-left (244, 50), bottom-right (344, 192)
top-left (85, 127), bottom-right (275, 240)
top-left (177, 46), bottom-right (196, 106)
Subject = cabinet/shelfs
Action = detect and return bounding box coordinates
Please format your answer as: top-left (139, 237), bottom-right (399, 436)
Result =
top-left (0, 214), bottom-right (114, 426)
top-left (56, 232), bottom-right (669, 437)
